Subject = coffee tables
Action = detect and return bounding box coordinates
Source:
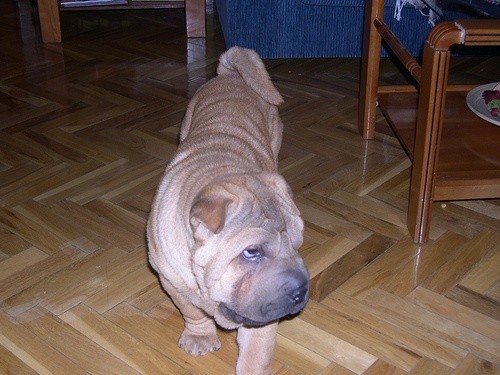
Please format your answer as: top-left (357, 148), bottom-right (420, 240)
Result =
top-left (360, 0), bottom-right (500, 244)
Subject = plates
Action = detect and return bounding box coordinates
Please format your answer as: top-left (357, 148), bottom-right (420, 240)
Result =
top-left (466, 82), bottom-right (500, 126)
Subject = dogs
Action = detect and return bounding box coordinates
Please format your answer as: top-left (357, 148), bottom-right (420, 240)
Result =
top-left (145, 45), bottom-right (310, 375)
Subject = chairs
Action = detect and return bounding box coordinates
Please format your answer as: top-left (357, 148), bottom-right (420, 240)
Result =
top-left (36, 0), bottom-right (207, 44)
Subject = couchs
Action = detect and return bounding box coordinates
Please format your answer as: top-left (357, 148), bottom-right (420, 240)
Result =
top-left (215, 0), bottom-right (470, 59)
